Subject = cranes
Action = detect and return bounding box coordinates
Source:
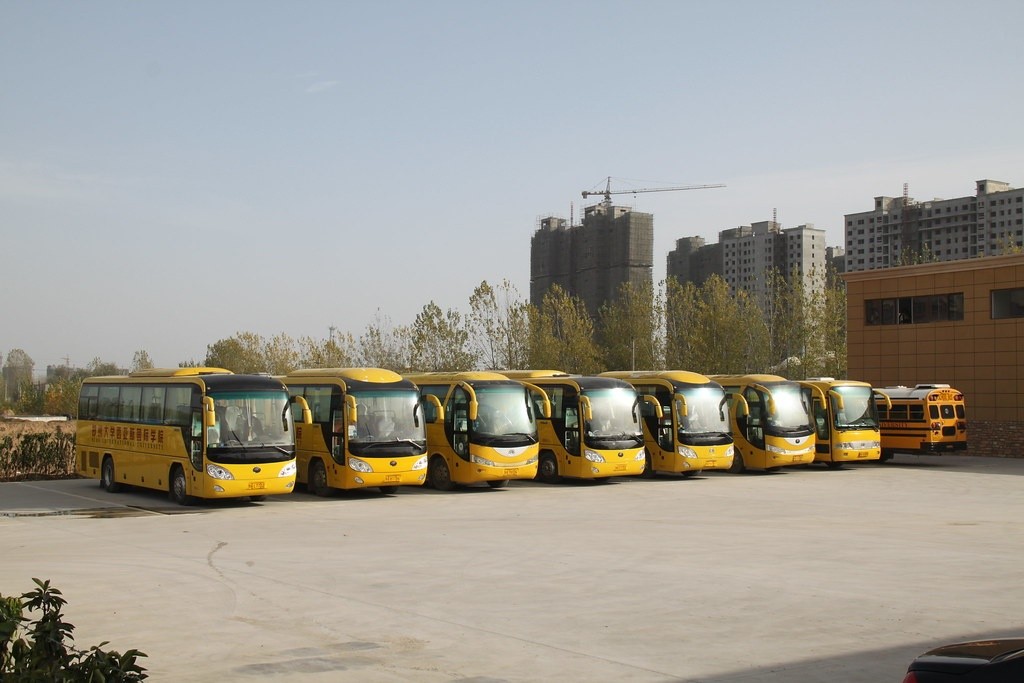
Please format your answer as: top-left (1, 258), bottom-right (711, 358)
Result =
top-left (581, 176), bottom-right (727, 206)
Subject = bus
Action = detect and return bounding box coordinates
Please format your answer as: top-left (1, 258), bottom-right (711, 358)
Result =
top-left (256, 368), bottom-right (444, 496)
top-left (704, 374), bottom-right (826, 474)
top-left (497, 370), bottom-right (663, 483)
top-left (76, 367), bottom-right (313, 505)
top-left (794, 377), bottom-right (892, 469)
top-left (595, 371), bottom-right (750, 479)
top-left (400, 371), bottom-right (552, 490)
top-left (872, 383), bottom-right (968, 458)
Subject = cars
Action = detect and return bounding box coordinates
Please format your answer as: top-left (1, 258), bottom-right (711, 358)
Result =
top-left (903, 638), bottom-right (1024, 683)
